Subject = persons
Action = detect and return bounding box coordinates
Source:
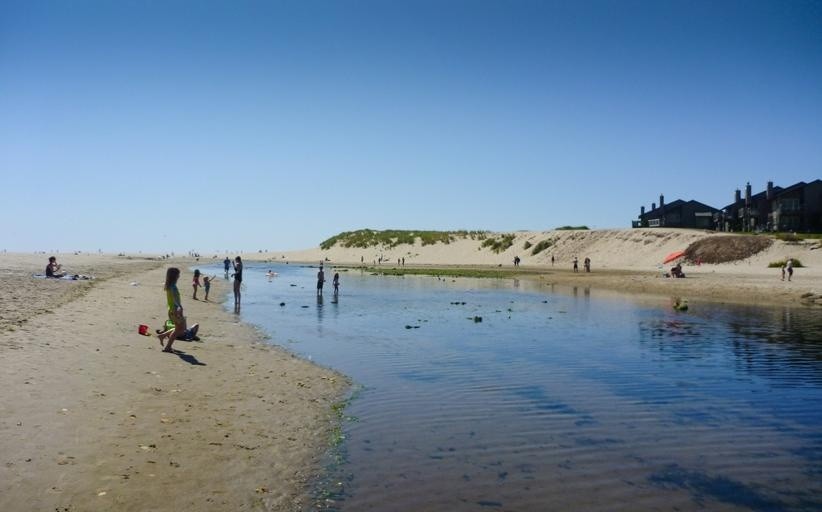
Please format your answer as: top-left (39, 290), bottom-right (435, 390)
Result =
top-left (266, 268), bottom-right (276, 276)
top-left (786, 258), bottom-right (793, 281)
top-left (511, 257), bottom-right (517, 266)
top-left (585, 256), bottom-right (590, 272)
top-left (224, 256), bottom-right (231, 272)
top-left (46, 256), bottom-right (64, 278)
top-left (515, 256), bottom-right (520, 264)
top-left (157, 267), bottom-right (186, 352)
top-left (572, 257), bottom-right (578, 272)
top-left (333, 273), bottom-right (339, 292)
top-left (203, 275), bottom-right (215, 300)
top-left (670, 263), bottom-right (681, 278)
top-left (231, 255), bottom-right (242, 303)
top-left (164, 307), bottom-right (200, 341)
top-left (316, 266), bottom-right (324, 293)
top-left (192, 269), bottom-right (201, 300)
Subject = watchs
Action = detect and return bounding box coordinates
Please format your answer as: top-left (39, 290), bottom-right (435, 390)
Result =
top-left (779, 262), bottom-right (785, 281)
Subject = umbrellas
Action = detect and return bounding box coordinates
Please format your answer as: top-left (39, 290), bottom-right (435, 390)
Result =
top-left (663, 251), bottom-right (685, 265)
